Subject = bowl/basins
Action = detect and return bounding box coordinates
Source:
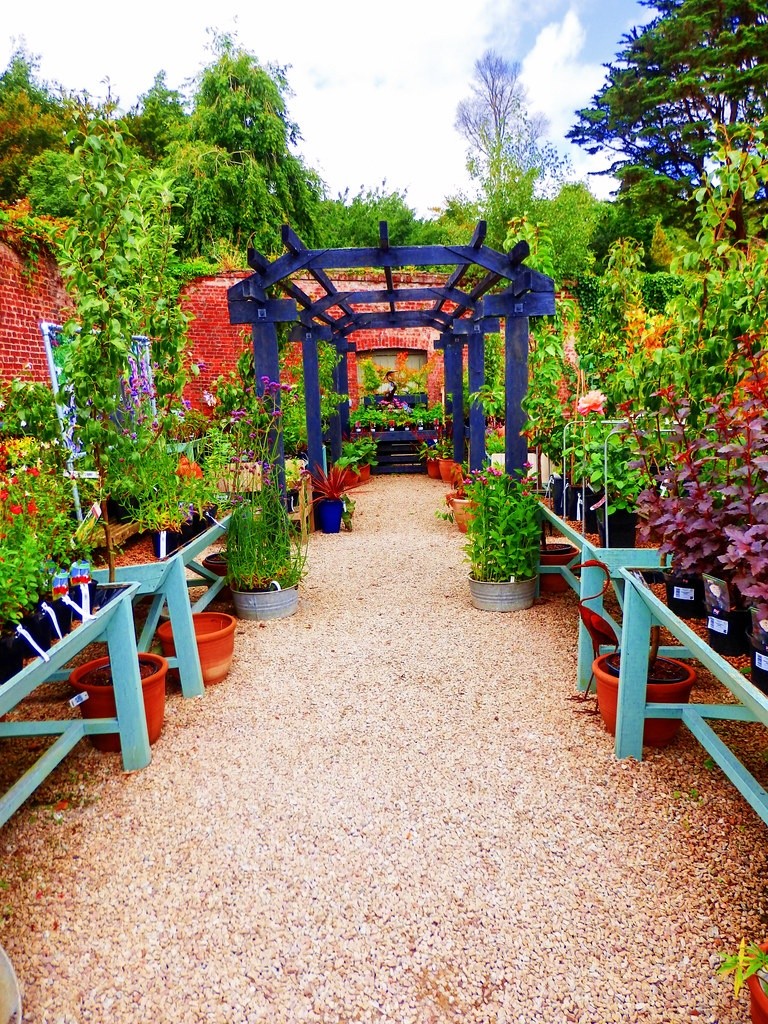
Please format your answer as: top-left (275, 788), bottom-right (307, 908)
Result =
top-left (159, 613), bottom-right (236, 686)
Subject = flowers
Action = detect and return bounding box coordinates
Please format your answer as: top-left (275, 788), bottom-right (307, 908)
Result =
top-left (84, 350), bottom-right (222, 532)
top-left (0, 420), bottom-right (95, 625)
top-left (460, 461), bottom-right (543, 583)
top-left (562, 390), bottom-right (646, 516)
top-left (224, 377), bottom-right (310, 592)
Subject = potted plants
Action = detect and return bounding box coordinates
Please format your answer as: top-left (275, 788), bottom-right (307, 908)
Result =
top-left (334, 434), bottom-right (379, 486)
top-left (715, 936), bottom-right (768, 1024)
top-left (413, 115), bottom-right (768, 693)
top-left (347, 403), bottom-right (444, 432)
top-left (306, 462), bottom-right (373, 534)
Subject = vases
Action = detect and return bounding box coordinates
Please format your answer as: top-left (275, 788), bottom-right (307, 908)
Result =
top-left (68, 651), bottom-right (168, 753)
top-left (0, 578), bottom-right (99, 686)
top-left (107, 478), bottom-right (162, 523)
top-left (151, 502), bottom-right (219, 559)
top-left (229, 579), bottom-right (298, 621)
top-left (158, 613), bottom-right (236, 685)
top-left (203, 552), bottom-right (232, 600)
top-left (595, 508), bottom-right (638, 548)
top-left (540, 548), bottom-right (579, 594)
top-left (465, 570), bottom-right (537, 613)
top-left (591, 651), bottom-right (695, 748)
